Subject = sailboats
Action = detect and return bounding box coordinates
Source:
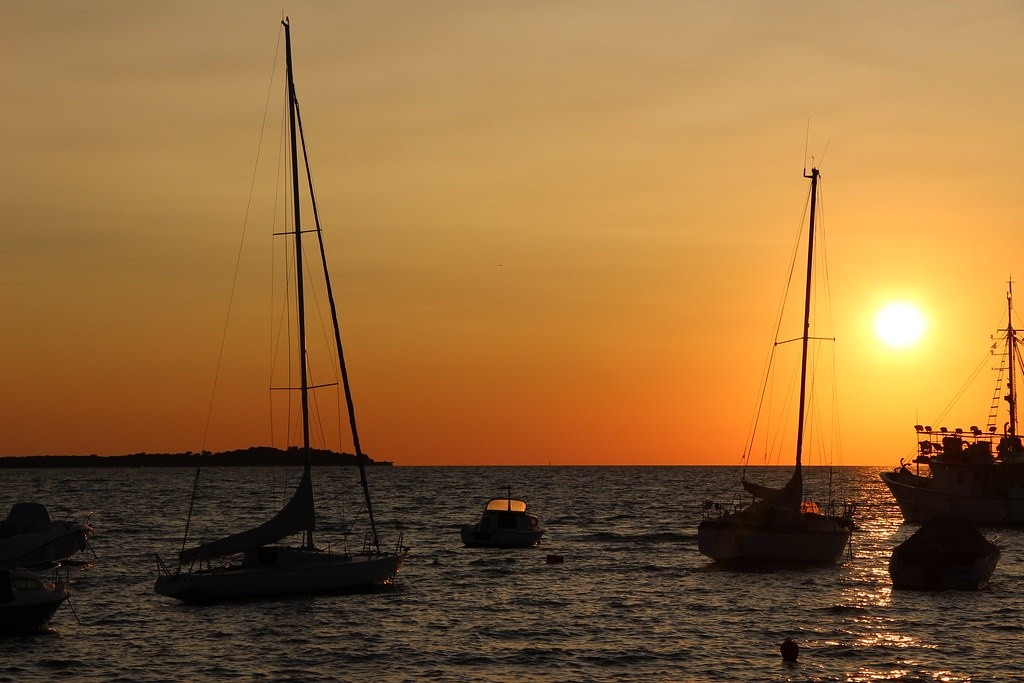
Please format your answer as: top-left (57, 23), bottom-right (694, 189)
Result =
top-left (152, 9), bottom-right (417, 599)
top-left (694, 156), bottom-right (863, 566)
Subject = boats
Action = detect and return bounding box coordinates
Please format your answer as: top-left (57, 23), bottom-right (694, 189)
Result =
top-left (886, 508), bottom-right (1004, 591)
top-left (1, 556), bottom-right (73, 642)
top-left (461, 481), bottom-right (551, 550)
top-left (1, 501), bottom-right (98, 570)
top-left (877, 274), bottom-right (1024, 533)
